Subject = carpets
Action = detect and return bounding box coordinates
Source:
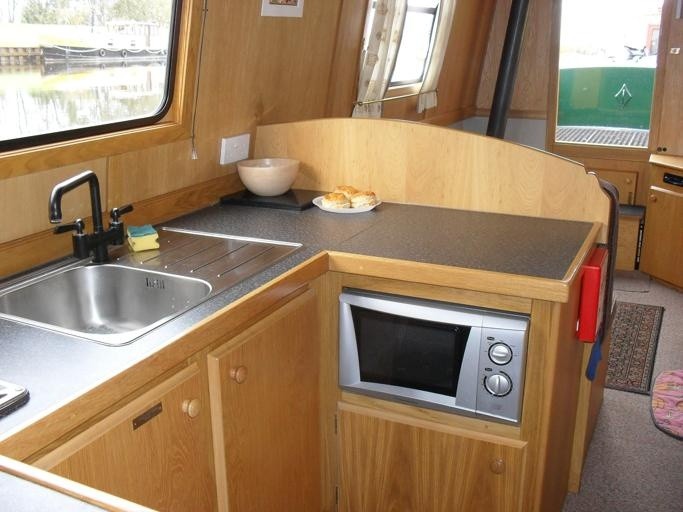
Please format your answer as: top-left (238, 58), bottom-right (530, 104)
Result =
top-left (602, 301), bottom-right (664, 396)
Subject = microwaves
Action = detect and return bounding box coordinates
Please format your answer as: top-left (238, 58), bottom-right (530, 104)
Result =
top-left (340, 292), bottom-right (530, 426)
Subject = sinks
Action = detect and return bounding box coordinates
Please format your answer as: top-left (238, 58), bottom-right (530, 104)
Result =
top-left (0, 260), bottom-right (212, 349)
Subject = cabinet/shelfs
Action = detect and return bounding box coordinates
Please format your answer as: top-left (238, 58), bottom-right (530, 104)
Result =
top-left (31, 287), bottom-right (323, 512)
top-left (639, 186), bottom-right (683, 290)
top-left (333, 404), bottom-right (526, 512)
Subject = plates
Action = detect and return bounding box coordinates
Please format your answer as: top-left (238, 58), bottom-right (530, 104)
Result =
top-left (310, 195), bottom-right (382, 214)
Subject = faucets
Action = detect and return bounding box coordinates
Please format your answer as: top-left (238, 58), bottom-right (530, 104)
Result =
top-left (49, 168), bottom-right (134, 267)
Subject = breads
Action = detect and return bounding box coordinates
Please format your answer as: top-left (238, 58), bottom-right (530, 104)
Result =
top-left (351, 191), bottom-right (377, 208)
top-left (323, 193), bottom-right (349, 209)
top-left (334, 184), bottom-right (359, 200)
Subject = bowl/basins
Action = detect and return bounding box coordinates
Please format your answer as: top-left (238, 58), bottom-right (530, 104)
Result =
top-left (237, 157), bottom-right (299, 197)
